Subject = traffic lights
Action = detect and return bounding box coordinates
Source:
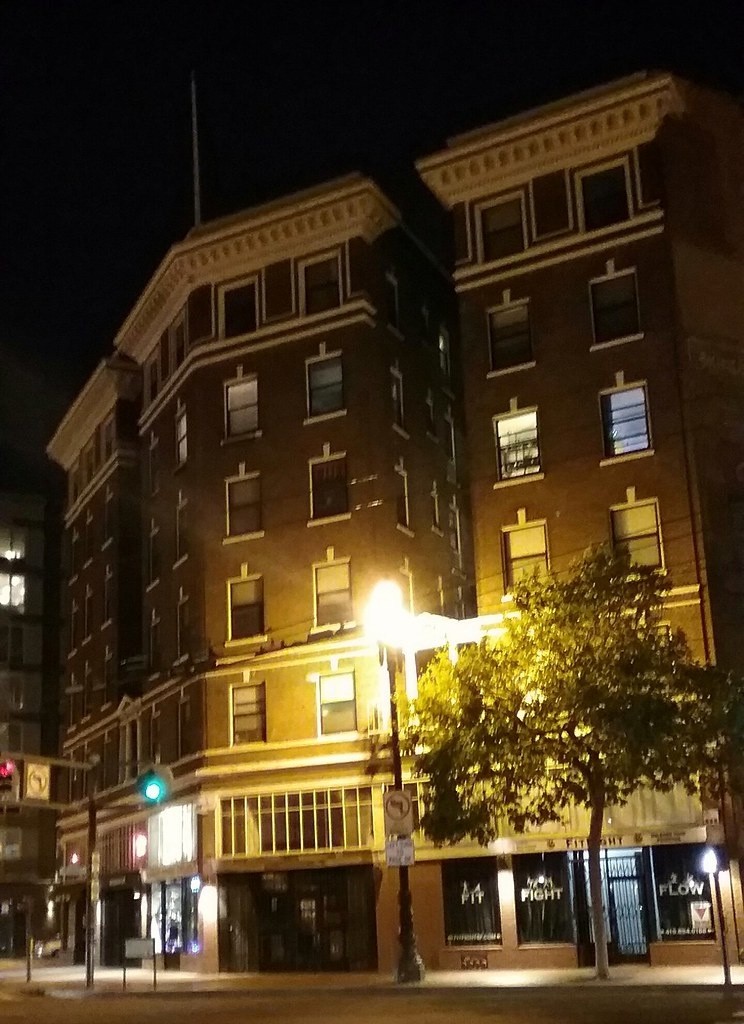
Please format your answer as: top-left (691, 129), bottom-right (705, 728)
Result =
top-left (145, 782), bottom-right (162, 803)
top-left (0, 760), bottom-right (17, 778)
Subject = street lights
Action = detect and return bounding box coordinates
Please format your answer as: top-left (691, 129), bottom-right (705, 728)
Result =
top-left (364, 579), bottom-right (427, 982)
